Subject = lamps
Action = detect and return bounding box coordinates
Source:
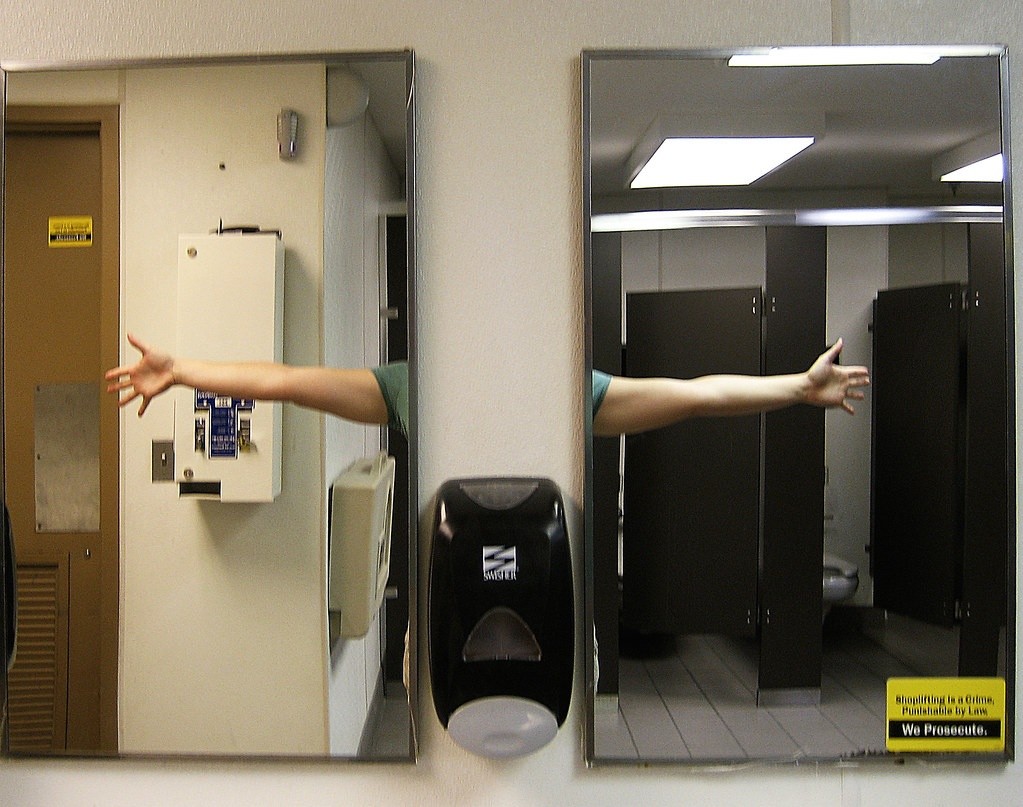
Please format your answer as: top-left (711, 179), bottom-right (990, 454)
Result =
top-left (618, 103), bottom-right (829, 188)
top-left (930, 127), bottom-right (1003, 189)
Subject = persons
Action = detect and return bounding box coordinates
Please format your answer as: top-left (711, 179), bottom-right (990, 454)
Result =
top-left (105, 334), bottom-right (869, 440)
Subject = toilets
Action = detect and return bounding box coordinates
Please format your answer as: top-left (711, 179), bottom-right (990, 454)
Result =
top-left (822, 551), bottom-right (860, 605)
